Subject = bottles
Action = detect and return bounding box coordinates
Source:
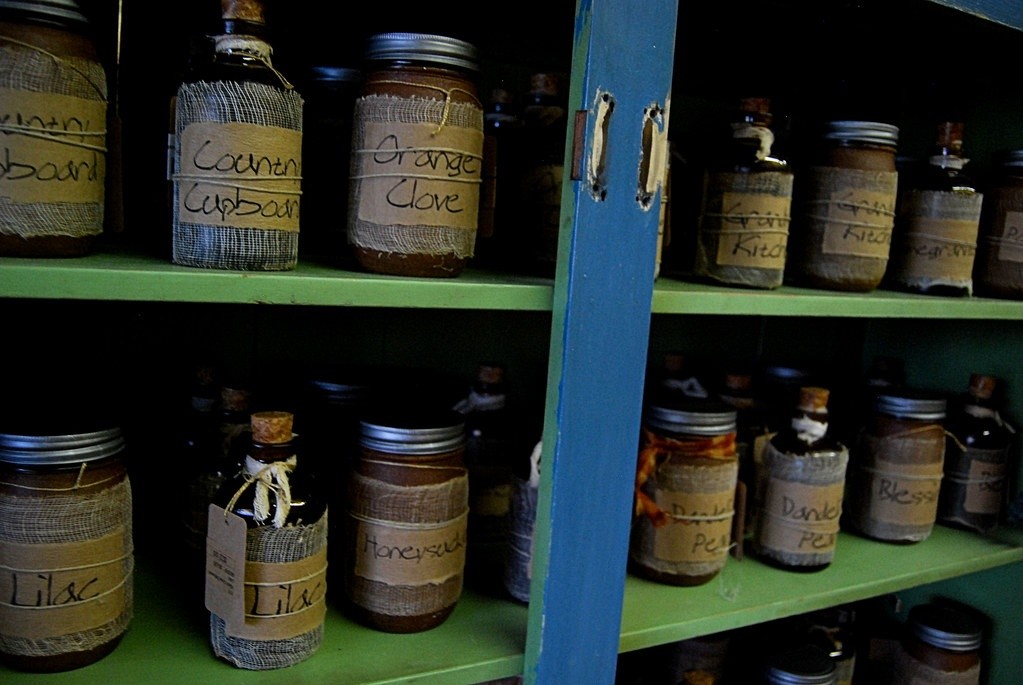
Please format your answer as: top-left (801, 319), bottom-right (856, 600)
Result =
top-left (898, 119), bottom-right (983, 297)
top-left (455, 360), bottom-right (525, 550)
top-left (173, 0), bottom-right (302, 271)
top-left (204, 410), bottom-right (332, 672)
top-left (941, 374), bottom-right (1007, 532)
top-left (754, 385), bottom-right (847, 573)
top-left (698, 95), bottom-right (798, 288)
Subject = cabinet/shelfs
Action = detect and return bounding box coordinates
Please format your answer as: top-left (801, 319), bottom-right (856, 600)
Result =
top-left (1, 0), bottom-right (1023, 684)
